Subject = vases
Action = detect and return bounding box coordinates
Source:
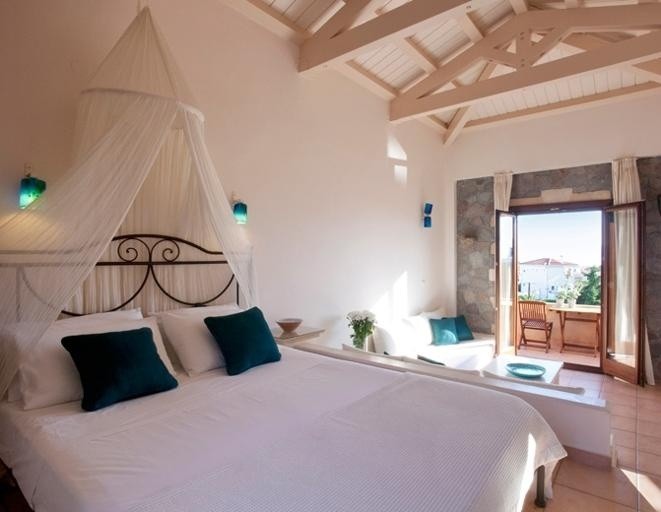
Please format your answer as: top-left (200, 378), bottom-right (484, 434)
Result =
top-left (567, 298), bottom-right (577, 308)
top-left (556, 297), bottom-right (565, 307)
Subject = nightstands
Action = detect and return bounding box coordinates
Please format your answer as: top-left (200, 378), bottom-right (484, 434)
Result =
top-left (269, 325), bottom-right (324, 347)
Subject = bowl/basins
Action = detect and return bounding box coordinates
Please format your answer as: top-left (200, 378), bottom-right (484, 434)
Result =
top-left (276, 318), bottom-right (303, 332)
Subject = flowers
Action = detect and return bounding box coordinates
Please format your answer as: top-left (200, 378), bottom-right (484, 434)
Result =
top-left (548, 266), bottom-right (589, 298)
top-left (345, 311), bottom-right (382, 351)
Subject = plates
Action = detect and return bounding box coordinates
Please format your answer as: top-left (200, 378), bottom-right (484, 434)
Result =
top-left (505, 363), bottom-right (546, 378)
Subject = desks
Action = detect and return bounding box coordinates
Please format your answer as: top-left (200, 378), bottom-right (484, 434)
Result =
top-left (549, 306), bottom-right (602, 357)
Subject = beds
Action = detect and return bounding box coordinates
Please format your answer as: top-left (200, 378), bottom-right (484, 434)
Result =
top-left (0, 234), bottom-right (565, 512)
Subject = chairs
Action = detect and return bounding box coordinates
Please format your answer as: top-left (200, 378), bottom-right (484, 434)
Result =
top-left (517, 301), bottom-right (553, 354)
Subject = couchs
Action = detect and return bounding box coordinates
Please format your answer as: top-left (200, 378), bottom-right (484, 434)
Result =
top-left (374, 308), bottom-right (496, 369)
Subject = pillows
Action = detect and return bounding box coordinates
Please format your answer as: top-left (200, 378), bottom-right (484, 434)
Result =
top-left (5, 303), bottom-right (282, 412)
top-left (442, 315), bottom-right (475, 340)
top-left (429, 318), bottom-right (460, 345)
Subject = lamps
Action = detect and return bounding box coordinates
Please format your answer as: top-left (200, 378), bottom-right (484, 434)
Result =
top-left (233, 192), bottom-right (248, 224)
top-left (422, 202), bottom-right (433, 228)
top-left (18, 163), bottom-right (49, 210)
